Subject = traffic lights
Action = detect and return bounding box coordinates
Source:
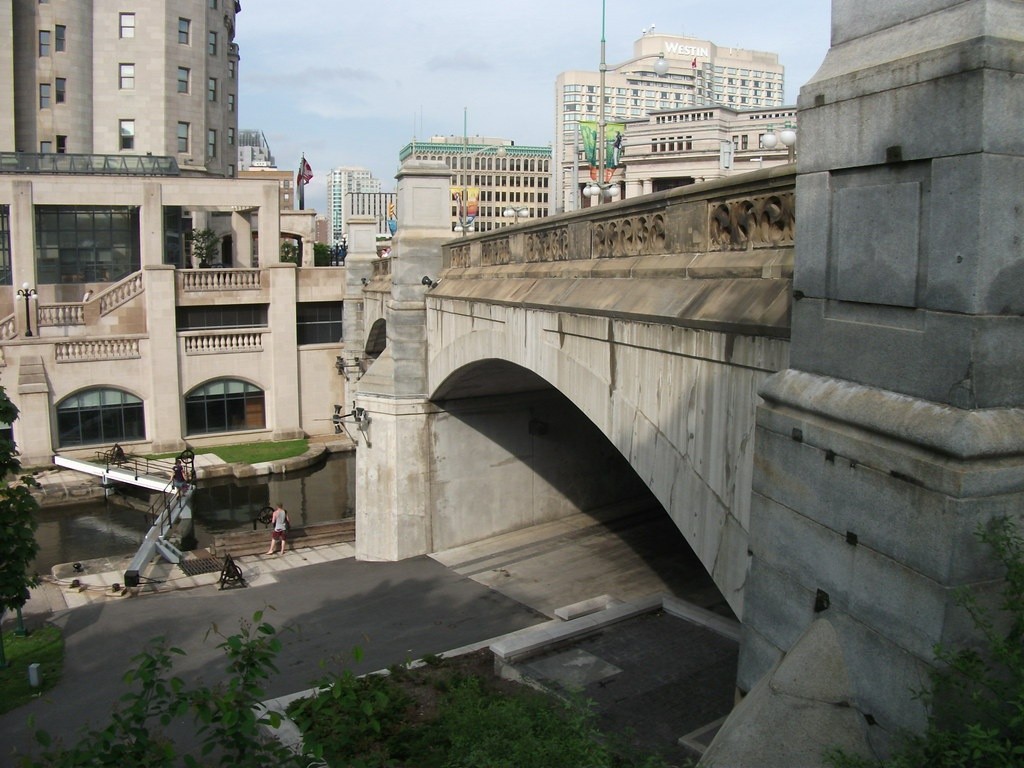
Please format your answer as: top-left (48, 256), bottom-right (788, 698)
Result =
top-left (388, 204), bottom-right (395, 218)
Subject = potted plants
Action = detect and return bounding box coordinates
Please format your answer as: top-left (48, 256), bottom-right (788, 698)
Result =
top-left (190, 228), bottom-right (220, 268)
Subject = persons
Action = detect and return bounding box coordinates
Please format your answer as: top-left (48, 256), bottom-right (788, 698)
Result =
top-left (82, 290), bottom-right (93, 302)
top-left (266, 504), bottom-right (289, 555)
top-left (170, 460), bottom-right (186, 499)
top-left (381, 247), bottom-right (392, 257)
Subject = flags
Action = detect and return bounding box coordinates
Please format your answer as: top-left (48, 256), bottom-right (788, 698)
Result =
top-left (297, 157), bottom-right (313, 184)
top-left (692, 58), bottom-right (696, 68)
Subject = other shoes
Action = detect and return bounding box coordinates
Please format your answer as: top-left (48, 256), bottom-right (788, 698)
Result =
top-left (266, 551), bottom-right (273, 555)
top-left (281, 551), bottom-right (285, 555)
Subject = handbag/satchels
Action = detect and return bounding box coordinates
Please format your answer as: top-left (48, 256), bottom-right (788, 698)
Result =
top-left (284, 509), bottom-right (290, 529)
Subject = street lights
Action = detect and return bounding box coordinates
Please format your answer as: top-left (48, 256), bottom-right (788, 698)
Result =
top-left (335, 240), bottom-right (340, 266)
top-left (342, 234), bottom-right (348, 262)
top-left (330, 245), bottom-right (333, 267)
top-left (583, 37), bottom-right (669, 200)
top-left (460, 144), bottom-right (507, 235)
